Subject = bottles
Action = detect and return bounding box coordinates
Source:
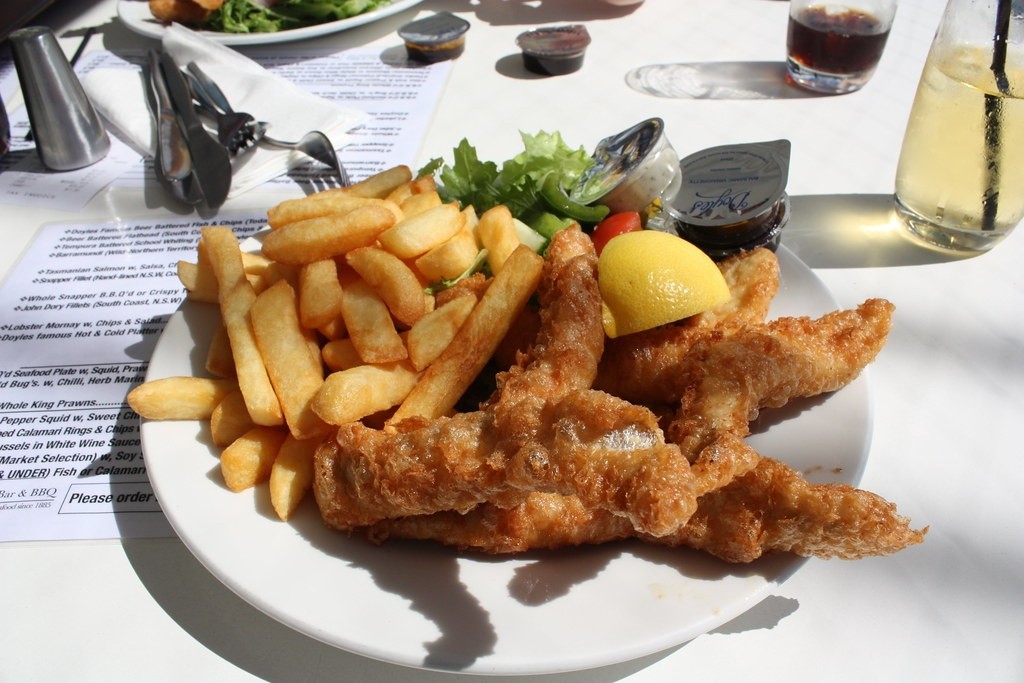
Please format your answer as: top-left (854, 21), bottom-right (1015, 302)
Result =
top-left (10, 25), bottom-right (112, 171)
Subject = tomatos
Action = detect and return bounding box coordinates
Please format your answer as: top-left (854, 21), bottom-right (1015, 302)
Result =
top-left (594, 211), bottom-right (640, 255)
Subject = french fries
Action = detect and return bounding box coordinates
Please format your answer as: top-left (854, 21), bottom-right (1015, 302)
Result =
top-left (128, 165), bottom-right (546, 520)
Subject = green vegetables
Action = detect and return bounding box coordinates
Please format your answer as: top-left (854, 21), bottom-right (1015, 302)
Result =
top-left (419, 131), bottom-right (610, 293)
top-left (211, 0), bottom-right (386, 34)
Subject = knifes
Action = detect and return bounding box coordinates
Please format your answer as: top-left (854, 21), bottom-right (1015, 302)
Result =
top-left (145, 49), bottom-right (231, 209)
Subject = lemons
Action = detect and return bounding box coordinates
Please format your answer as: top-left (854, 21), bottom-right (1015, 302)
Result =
top-left (600, 231), bottom-right (732, 335)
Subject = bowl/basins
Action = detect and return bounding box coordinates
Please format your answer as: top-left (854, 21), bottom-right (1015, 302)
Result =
top-left (670, 148), bottom-right (791, 264)
top-left (398, 13), bottom-right (470, 61)
top-left (516, 24), bottom-right (591, 74)
top-left (568, 117), bottom-right (681, 218)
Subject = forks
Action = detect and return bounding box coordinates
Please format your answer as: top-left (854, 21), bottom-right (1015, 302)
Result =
top-left (181, 61), bottom-right (354, 191)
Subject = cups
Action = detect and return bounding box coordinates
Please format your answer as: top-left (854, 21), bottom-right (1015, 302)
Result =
top-left (895, 0), bottom-right (1024, 259)
top-left (788, 0), bottom-right (898, 97)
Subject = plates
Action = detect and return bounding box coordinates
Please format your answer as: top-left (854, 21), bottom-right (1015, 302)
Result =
top-left (116, 0), bottom-right (423, 46)
top-left (139, 222), bottom-right (878, 678)
